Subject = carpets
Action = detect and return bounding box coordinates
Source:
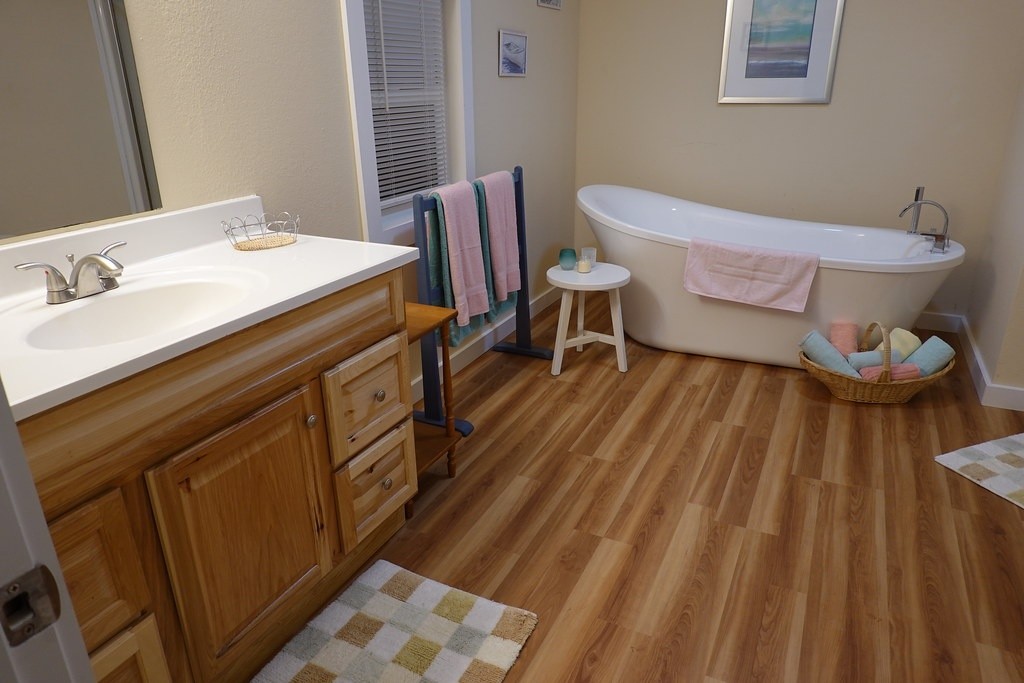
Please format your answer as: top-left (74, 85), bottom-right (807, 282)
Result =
top-left (249, 559), bottom-right (539, 683)
top-left (935, 432), bottom-right (1024, 510)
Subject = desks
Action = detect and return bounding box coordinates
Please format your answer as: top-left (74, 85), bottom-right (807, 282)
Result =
top-left (405, 301), bottom-right (463, 518)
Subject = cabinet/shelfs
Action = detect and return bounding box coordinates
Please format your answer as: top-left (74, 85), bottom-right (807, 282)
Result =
top-left (13, 265), bottom-right (418, 682)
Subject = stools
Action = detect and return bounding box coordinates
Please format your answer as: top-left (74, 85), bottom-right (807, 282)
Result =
top-left (547, 262), bottom-right (630, 375)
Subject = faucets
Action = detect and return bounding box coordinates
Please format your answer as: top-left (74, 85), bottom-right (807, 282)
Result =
top-left (899, 198), bottom-right (951, 254)
top-left (67, 253), bottom-right (124, 301)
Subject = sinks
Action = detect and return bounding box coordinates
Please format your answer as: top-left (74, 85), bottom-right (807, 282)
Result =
top-left (24, 280), bottom-right (249, 351)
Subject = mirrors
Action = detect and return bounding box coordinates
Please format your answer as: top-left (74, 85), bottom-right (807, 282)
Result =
top-left (0, 0), bottom-right (162, 240)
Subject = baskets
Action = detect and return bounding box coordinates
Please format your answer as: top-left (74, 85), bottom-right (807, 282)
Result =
top-left (798, 322), bottom-right (955, 404)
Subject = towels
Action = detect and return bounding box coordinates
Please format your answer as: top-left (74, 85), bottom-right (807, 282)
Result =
top-left (798, 321), bottom-right (957, 381)
top-left (683, 236), bottom-right (821, 314)
top-left (427, 170), bottom-right (523, 350)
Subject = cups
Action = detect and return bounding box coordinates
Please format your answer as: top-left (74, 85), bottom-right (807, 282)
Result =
top-left (582, 247), bottom-right (596, 268)
top-left (559, 249), bottom-right (576, 271)
top-left (579, 256), bottom-right (591, 273)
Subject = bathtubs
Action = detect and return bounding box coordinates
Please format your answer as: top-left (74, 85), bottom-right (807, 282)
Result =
top-left (576, 183), bottom-right (968, 373)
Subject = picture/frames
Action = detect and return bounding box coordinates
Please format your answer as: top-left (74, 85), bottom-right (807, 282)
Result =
top-left (499, 29), bottom-right (528, 76)
top-left (537, 0), bottom-right (562, 9)
top-left (717, 0), bottom-right (845, 103)
